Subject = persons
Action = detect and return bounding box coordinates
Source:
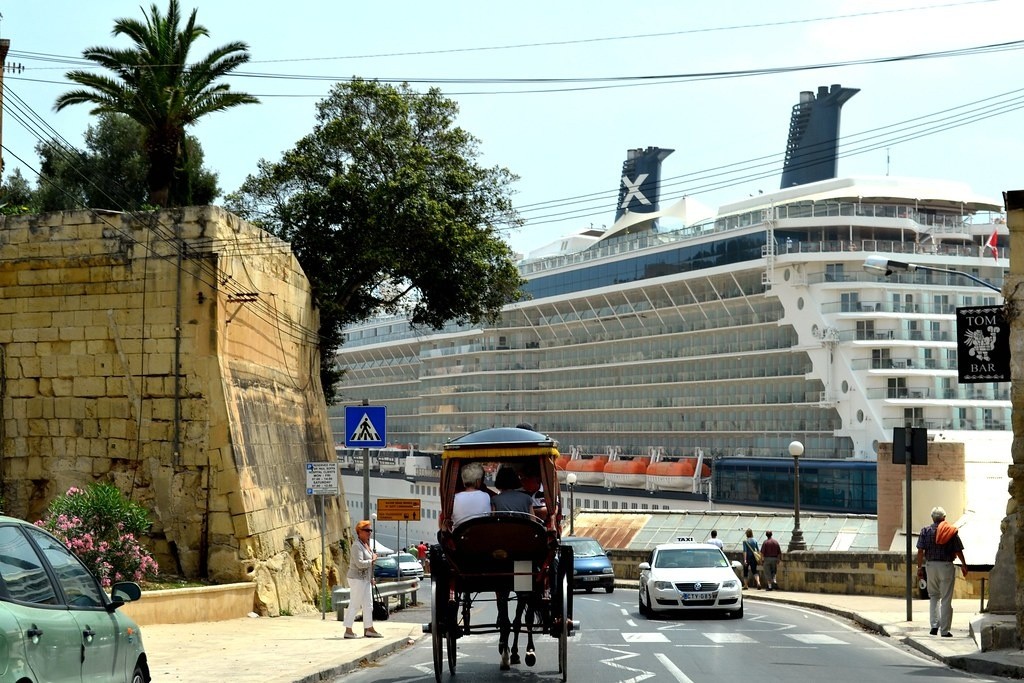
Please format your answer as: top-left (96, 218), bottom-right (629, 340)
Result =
top-left (742, 528), bottom-right (762, 590)
top-left (402, 541), bottom-right (431, 573)
top-left (491, 467), bottom-right (537, 522)
top-left (916, 507), bottom-right (968, 637)
top-left (760, 529), bottom-right (781, 591)
top-left (451, 463), bottom-right (491, 524)
top-left (707, 530), bottom-right (723, 550)
top-left (343, 520), bottom-right (383, 639)
top-left (786, 237), bottom-right (793, 253)
top-left (503, 423), bottom-right (559, 520)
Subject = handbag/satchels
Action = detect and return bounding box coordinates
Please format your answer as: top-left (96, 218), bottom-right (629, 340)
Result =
top-left (372, 601), bottom-right (389, 621)
top-left (753, 551), bottom-right (762, 562)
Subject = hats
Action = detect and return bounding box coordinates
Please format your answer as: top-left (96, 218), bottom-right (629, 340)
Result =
top-left (765, 530), bottom-right (772, 535)
top-left (744, 528), bottom-right (752, 534)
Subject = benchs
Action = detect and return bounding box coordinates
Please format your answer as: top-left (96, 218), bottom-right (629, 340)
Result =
top-left (451, 511), bottom-right (547, 559)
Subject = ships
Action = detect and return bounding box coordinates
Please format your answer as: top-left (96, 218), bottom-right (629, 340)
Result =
top-left (322, 85), bottom-right (1011, 554)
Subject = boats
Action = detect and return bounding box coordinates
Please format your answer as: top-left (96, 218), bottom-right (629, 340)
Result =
top-left (378, 446), bottom-right (416, 472)
top-left (333, 443), bottom-right (355, 468)
top-left (351, 446), bottom-right (381, 471)
top-left (605, 455), bottom-right (650, 485)
top-left (647, 456), bottom-right (709, 488)
top-left (567, 455), bottom-right (608, 485)
top-left (481, 454), bottom-right (570, 482)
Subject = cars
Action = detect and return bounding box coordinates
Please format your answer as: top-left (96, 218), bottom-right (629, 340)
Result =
top-left (0, 513), bottom-right (152, 683)
top-left (639, 536), bottom-right (744, 618)
top-left (374, 556), bottom-right (401, 579)
top-left (556, 536), bottom-right (616, 594)
top-left (390, 551), bottom-right (425, 580)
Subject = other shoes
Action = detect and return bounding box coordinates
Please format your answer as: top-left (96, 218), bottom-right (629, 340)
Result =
top-left (766, 588), bottom-right (772, 591)
top-left (742, 587), bottom-right (748, 590)
top-left (757, 585), bottom-right (761, 590)
top-left (930, 628), bottom-right (953, 637)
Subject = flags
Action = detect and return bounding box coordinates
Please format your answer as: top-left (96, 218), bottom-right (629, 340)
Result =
top-left (986, 230), bottom-right (998, 264)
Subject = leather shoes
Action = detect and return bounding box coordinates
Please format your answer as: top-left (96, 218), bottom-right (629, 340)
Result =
top-left (365, 631), bottom-right (384, 638)
top-left (344, 632), bottom-right (362, 639)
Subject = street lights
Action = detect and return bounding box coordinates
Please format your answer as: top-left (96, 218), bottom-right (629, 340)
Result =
top-left (566, 474), bottom-right (578, 534)
top-left (787, 441), bottom-right (808, 552)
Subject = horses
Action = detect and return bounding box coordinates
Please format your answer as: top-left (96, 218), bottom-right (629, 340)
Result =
top-left (495, 592), bottom-right (538, 673)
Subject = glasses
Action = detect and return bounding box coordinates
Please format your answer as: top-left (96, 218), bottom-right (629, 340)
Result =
top-left (361, 529), bottom-right (372, 532)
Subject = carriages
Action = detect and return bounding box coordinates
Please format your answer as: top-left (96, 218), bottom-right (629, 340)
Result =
top-left (423, 426), bottom-right (580, 683)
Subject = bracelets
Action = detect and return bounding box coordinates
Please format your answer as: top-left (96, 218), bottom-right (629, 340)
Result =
top-left (918, 567), bottom-right (922, 570)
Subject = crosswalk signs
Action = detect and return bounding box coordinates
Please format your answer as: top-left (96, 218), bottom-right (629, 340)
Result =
top-left (345, 404), bottom-right (387, 448)
